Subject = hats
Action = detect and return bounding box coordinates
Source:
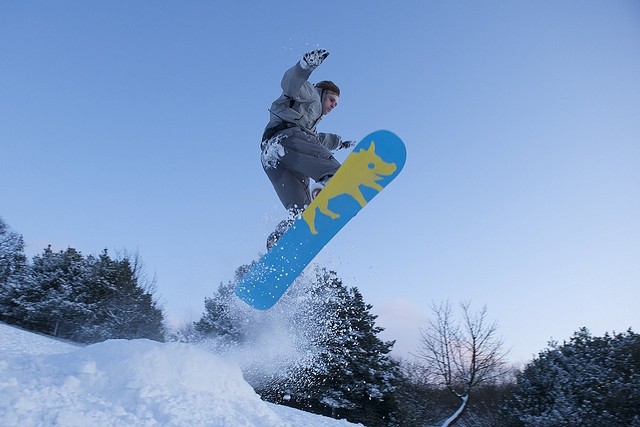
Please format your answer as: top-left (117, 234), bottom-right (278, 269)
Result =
top-left (316, 80), bottom-right (340, 96)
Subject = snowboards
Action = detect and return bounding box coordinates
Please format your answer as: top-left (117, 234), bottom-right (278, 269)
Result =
top-left (235, 128), bottom-right (406, 310)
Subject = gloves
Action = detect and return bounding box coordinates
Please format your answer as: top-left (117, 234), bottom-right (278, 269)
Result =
top-left (341, 140), bottom-right (355, 149)
top-left (300, 48), bottom-right (329, 69)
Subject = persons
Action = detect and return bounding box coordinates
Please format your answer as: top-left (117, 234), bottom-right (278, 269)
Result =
top-left (259, 49), bottom-right (356, 250)
